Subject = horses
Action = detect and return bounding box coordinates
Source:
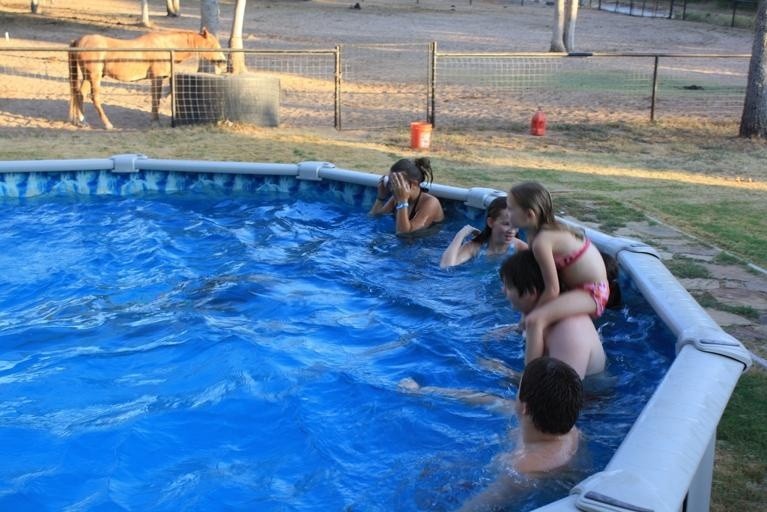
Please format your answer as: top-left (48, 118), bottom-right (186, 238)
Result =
top-left (67, 25), bottom-right (229, 131)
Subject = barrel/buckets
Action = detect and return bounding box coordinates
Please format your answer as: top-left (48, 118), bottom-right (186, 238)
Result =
top-left (530, 108), bottom-right (548, 135)
top-left (409, 121), bottom-right (433, 150)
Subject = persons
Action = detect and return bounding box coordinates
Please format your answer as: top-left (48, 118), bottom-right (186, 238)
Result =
top-left (368, 157), bottom-right (444, 236)
top-left (396, 355), bottom-right (584, 511)
top-left (475, 249), bottom-right (605, 387)
top-left (506, 180), bottom-right (611, 368)
top-left (439, 196), bottom-right (529, 270)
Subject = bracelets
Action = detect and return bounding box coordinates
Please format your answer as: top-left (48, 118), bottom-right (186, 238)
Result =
top-left (376, 196), bottom-right (385, 202)
top-left (396, 202), bottom-right (409, 211)
